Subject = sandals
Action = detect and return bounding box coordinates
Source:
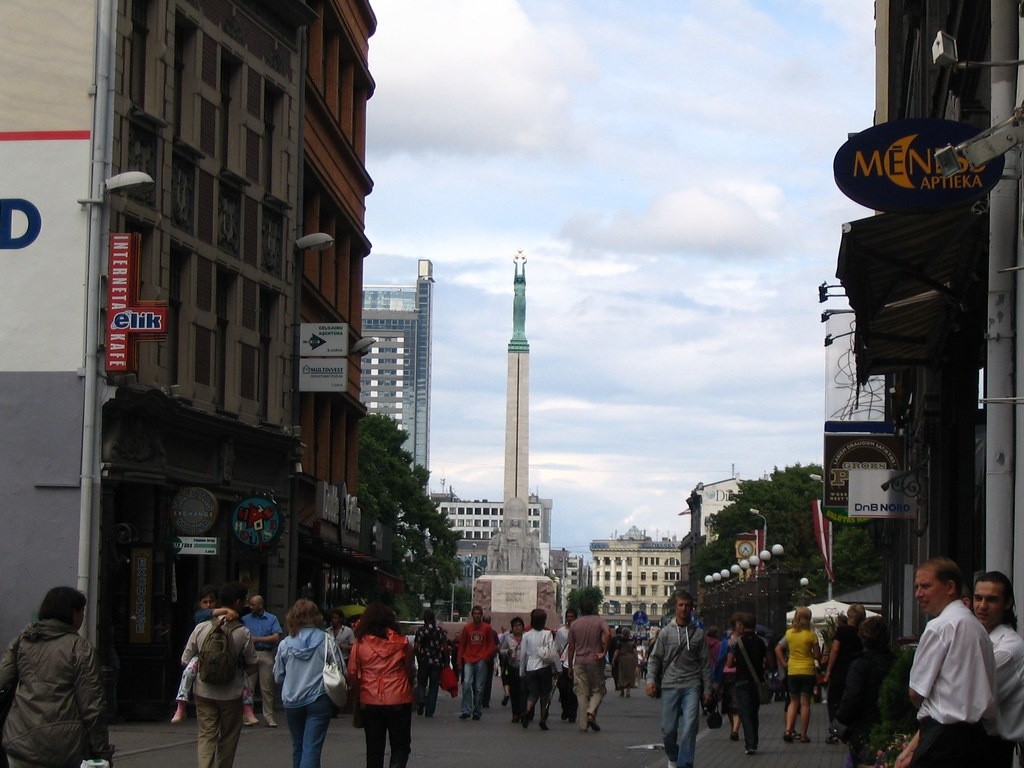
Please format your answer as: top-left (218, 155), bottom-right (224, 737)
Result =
top-left (783, 730), bottom-right (794, 742)
top-left (825, 736), bottom-right (839, 744)
top-left (800, 735), bottom-right (811, 742)
top-left (792, 731), bottom-right (801, 740)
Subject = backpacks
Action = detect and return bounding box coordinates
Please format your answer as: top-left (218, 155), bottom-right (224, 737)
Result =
top-left (198, 617), bottom-right (245, 685)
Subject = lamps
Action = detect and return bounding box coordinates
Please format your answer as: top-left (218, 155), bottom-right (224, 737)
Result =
top-left (350, 338), bottom-right (376, 355)
top-left (825, 332), bottom-right (855, 346)
top-left (294, 232), bottom-right (335, 253)
top-left (822, 309), bottom-right (856, 323)
top-left (76, 170), bottom-right (155, 211)
top-left (819, 281), bottom-right (846, 302)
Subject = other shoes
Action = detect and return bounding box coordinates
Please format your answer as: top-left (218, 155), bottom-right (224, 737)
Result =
top-left (667, 760), bottom-right (677, 768)
top-left (730, 731), bottom-right (738, 741)
top-left (459, 713), bottom-right (471, 719)
top-left (172, 710), bottom-right (186, 724)
top-left (472, 715), bottom-right (480, 720)
top-left (417, 701), bottom-right (425, 715)
top-left (482, 702), bottom-right (489, 708)
top-left (502, 694), bottom-right (510, 706)
top-left (246, 714), bottom-right (259, 724)
top-left (264, 715), bottom-right (278, 727)
top-left (244, 714), bottom-right (251, 725)
top-left (512, 706), bottom-right (601, 733)
top-left (425, 711), bottom-right (433, 718)
top-left (744, 745), bottom-right (755, 754)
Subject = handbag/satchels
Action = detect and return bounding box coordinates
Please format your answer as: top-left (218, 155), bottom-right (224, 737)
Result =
top-left (352, 686), bottom-right (364, 729)
top-left (322, 631), bottom-right (347, 707)
top-left (707, 712), bottom-right (722, 729)
top-left (441, 665), bottom-right (458, 691)
top-left (654, 666), bottom-right (666, 698)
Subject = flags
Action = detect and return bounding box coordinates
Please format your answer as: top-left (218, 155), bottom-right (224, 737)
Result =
top-left (750, 529), bottom-right (766, 578)
top-left (811, 499), bottom-right (834, 582)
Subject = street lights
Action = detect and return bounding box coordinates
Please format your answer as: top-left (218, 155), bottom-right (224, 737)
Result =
top-left (749, 508), bottom-right (767, 568)
top-left (809, 474), bottom-right (833, 601)
top-left (471, 542), bottom-right (477, 611)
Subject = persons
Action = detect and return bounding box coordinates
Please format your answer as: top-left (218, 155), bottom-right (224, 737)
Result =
top-left (0, 586), bottom-right (115, 768)
top-left (172, 585), bottom-right (893, 768)
top-left (895, 557), bottom-right (1024, 768)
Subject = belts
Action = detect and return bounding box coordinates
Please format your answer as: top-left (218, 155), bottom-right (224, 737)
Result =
top-left (254, 646), bottom-right (274, 652)
